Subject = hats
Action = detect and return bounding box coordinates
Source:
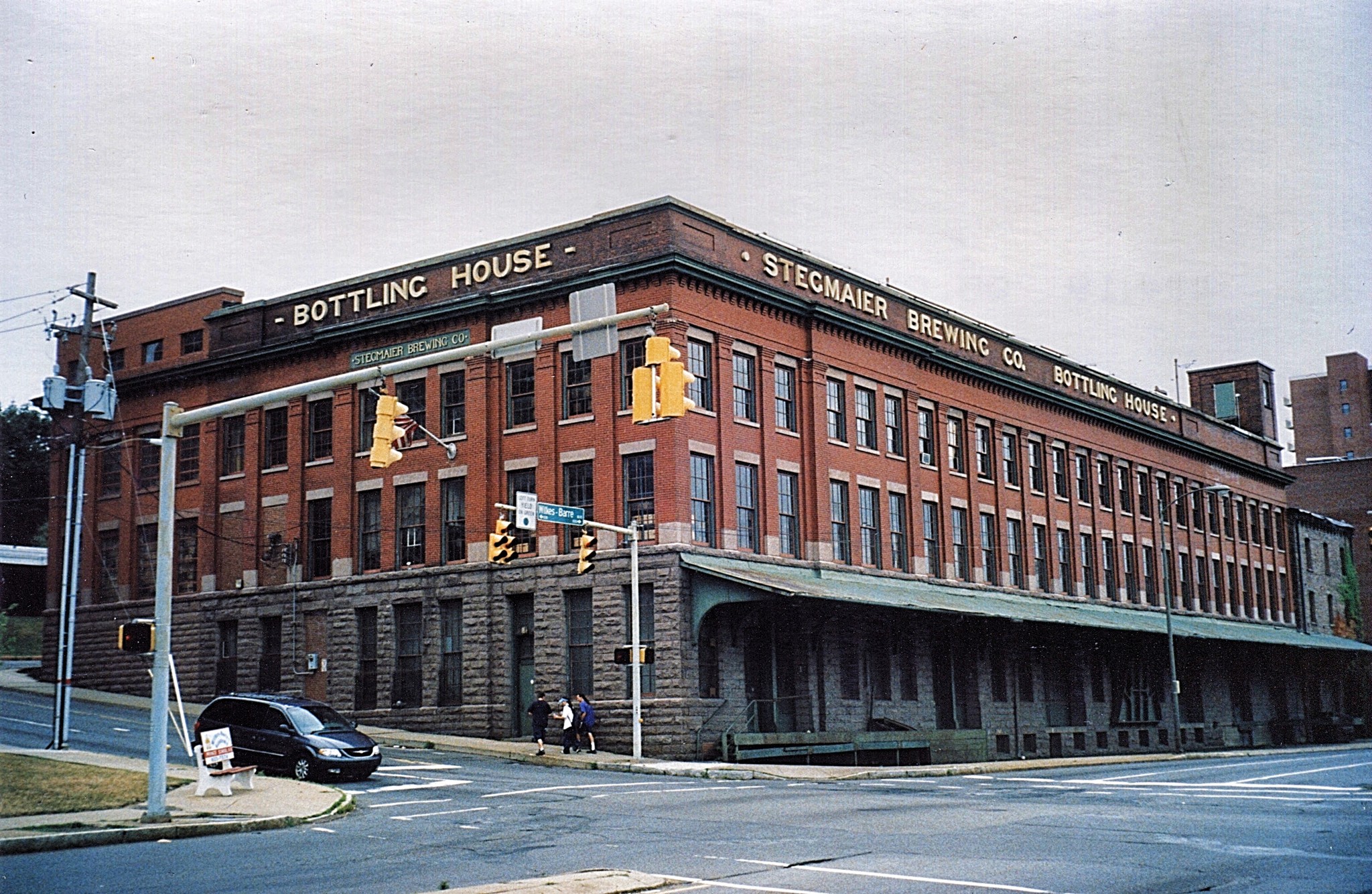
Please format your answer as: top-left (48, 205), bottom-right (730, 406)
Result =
top-left (559, 697), bottom-right (568, 703)
top-left (583, 703), bottom-right (595, 727)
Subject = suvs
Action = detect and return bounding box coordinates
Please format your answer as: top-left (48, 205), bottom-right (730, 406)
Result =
top-left (189, 692), bottom-right (382, 784)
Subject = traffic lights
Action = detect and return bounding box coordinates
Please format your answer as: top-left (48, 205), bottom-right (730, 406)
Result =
top-left (486, 519), bottom-right (519, 566)
top-left (613, 648), bottom-right (631, 665)
top-left (120, 622), bottom-right (156, 654)
top-left (639, 648), bottom-right (654, 664)
top-left (368, 395), bottom-right (408, 469)
top-left (632, 336), bottom-right (697, 424)
top-left (577, 535), bottom-right (597, 575)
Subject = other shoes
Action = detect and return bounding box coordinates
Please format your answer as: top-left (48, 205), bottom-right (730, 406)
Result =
top-left (586, 749), bottom-right (596, 754)
top-left (535, 749), bottom-right (545, 756)
top-left (576, 749), bottom-right (581, 753)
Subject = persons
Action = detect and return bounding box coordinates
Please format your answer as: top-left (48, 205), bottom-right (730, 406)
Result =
top-left (527, 691), bottom-right (553, 755)
top-left (552, 694), bottom-right (597, 755)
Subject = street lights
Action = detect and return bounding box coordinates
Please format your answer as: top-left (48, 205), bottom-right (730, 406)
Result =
top-left (1159, 485), bottom-right (1230, 755)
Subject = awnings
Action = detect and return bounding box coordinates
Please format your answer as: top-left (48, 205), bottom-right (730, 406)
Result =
top-left (677, 552), bottom-right (1372, 650)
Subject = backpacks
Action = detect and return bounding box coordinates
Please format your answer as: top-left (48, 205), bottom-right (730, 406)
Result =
top-left (538, 702), bottom-right (549, 724)
top-left (566, 705), bottom-right (581, 732)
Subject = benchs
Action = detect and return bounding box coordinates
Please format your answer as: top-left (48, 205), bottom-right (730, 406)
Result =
top-left (194, 743), bottom-right (258, 799)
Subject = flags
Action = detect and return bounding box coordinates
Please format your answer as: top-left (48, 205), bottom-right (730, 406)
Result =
top-left (391, 413), bottom-right (419, 449)
